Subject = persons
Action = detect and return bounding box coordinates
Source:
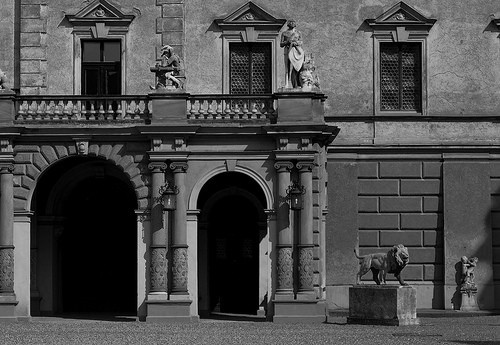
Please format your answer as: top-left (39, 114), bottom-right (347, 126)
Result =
top-left (279, 20), bottom-right (304, 89)
top-left (149, 49), bottom-right (183, 90)
top-left (466, 257), bottom-right (478, 283)
top-left (460, 256), bottom-right (471, 283)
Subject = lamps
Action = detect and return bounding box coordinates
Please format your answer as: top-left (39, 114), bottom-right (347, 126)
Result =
top-left (286, 180), bottom-right (306, 210)
top-left (158, 183), bottom-right (179, 211)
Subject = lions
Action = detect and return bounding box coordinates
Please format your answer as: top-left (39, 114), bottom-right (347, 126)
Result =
top-left (354, 244), bottom-right (409, 286)
